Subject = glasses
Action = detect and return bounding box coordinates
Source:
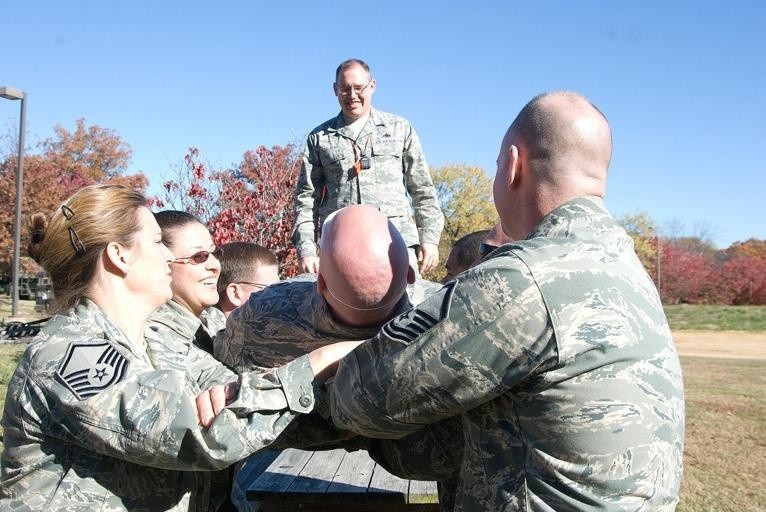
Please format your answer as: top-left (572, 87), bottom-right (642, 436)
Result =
top-left (171, 248), bottom-right (222, 266)
top-left (335, 79), bottom-right (372, 95)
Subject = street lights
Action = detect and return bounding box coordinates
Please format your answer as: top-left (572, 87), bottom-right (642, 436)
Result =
top-left (649, 225), bottom-right (662, 294)
top-left (0, 86), bottom-right (27, 319)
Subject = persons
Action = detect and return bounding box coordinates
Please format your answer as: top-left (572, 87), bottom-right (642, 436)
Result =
top-left (470, 221), bottom-right (514, 269)
top-left (213, 242), bottom-right (280, 320)
top-left (314, 90), bottom-right (685, 512)
top-left (146, 210), bottom-right (226, 355)
top-left (438, 230), bottom-right (491, 285)
top-left (0, 183), bottom-right (368, 512)
top-left (290, 59), bottom-right (444, 275)
top-left (213, 204), bottom-right (446, 375)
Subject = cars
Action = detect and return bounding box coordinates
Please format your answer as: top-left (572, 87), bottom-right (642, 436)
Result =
top-left (0, 271), bottom-right (53, 311)
